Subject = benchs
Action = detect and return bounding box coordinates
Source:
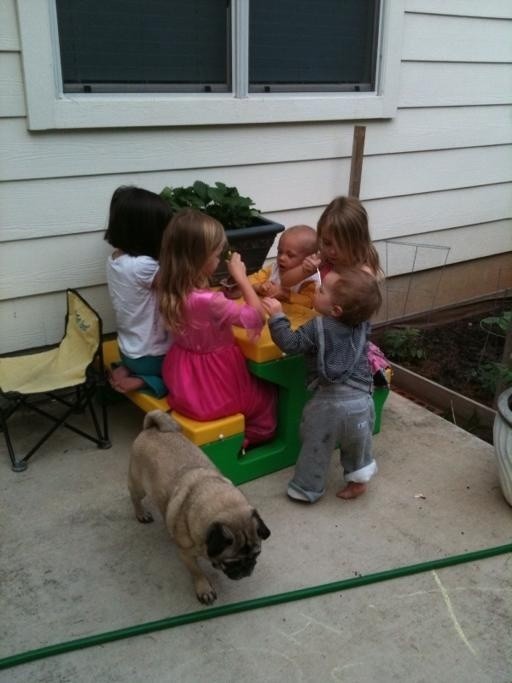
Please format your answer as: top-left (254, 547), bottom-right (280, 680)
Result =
top-left (374, 358), bottom-right (390, 436)
top-left (101, 340), bottom-right (249, 489)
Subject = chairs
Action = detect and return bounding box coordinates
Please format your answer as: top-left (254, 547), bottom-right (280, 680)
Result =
top-left (0, 286), bottom-right (112, 474)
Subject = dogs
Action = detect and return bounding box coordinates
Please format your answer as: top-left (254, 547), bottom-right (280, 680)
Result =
top-left (124, 408), bottom-right (271, 606)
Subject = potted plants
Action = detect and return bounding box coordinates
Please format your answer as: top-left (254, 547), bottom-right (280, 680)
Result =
top-left (157, 179), bottom-right (286, 288)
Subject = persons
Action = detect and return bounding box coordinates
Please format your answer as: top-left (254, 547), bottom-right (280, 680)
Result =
top-left (245, 224), bottom-right (321, 309)
top-left (260, 265), bottom-right (382, 504)
top-left (137, 207), bottom-right (279, 455)
top-left (280, 193), bottom-right (391, 374)
top-left (100, 184), bottom-right (175, 400)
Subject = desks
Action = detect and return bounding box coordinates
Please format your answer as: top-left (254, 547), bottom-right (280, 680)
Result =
top-left (160, 282), bottom-right (393, 486)
top-left (229, 293), bottom-right (319, 466)
